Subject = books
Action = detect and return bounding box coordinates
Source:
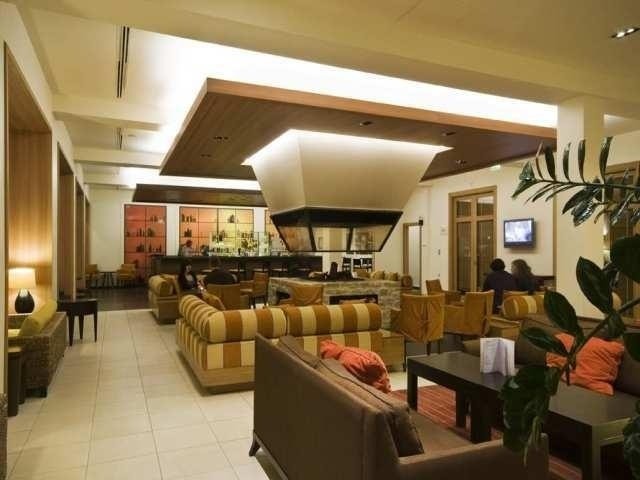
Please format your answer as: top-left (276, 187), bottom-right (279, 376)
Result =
top-left (479, 337), bottom-right (516, 378)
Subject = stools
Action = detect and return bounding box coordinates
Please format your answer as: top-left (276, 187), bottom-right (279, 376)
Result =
top-left (382, 331), bottom-right (405, 368)
top-left (57, 300), bottom-right (98, 346)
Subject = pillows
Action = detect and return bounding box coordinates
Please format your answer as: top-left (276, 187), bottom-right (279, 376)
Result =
top-left (276, 336), bottom-right (318, 369)
top-left (319, 339), bottom-right (390, 393)
top-left (546, 334), bottom-right (624, 396)
top-left (18, 300), bottom-right (57, 334)
top-left (316, 358), bottom-right (424, 455)
top-left (203, 292), bottom-right (224, 311)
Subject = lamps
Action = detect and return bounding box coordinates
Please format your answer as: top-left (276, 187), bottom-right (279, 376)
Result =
top-left (9, 268), bottom-right (36, 313)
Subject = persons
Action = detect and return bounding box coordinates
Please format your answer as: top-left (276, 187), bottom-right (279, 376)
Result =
top-left (511, 259), bottom-right (539, 293)
top-left (182, 241), bottom-right (193, 257)
top-left (179, 260), bottom-right (197, 290)
top-left (204, 262), bottom-right (235, 285)
top-left (484, 257), bottom-right (516, 314)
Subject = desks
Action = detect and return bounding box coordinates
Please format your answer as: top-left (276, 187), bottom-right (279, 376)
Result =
top-left (100, 271), bottom-right (116, 288)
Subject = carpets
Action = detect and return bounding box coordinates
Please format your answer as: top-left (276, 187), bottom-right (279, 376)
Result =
top-left (391, 385), bottom-right (583, 480)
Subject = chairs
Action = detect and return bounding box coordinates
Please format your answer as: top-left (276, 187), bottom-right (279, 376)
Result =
top-left (239, 272), bottom-right (268, 309)
top-left (117, 264), bottom-right (136, 288)
top-left (8, 311), bottom-right (67, 397)
top-left (390, 291), bottom-right (443, 371)
top-left (426, 279), bottom-right (463, 305)
top-left (443, 289), bottom-right (494, 341)
top-left (85, 264), bottom-right (98, 287)
top-left (200, 256), bottom-right (311, 284)
top-left (487, 291), bottom-right (544, 338)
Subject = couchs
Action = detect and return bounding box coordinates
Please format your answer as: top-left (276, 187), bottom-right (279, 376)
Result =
top-left (175, 294), bottom-right (384, 389)
top-left (462, 314), bottom-right (640, 395)
top-left (249, 332), bottom-right (548, 480)
top-left (147, 273), bottom-right (237, 325)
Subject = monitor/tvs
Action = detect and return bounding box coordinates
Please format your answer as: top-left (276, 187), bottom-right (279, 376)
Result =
top-left (503, 218), bottom-right (535, 247)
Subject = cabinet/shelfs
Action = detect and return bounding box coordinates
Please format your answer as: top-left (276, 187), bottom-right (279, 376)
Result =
top-left (265, 209), bottom-right (287, 256)
top-left (179, 206), bottom-right (254, 257)
top-left (124, 204), bottom-right (167, 287)
